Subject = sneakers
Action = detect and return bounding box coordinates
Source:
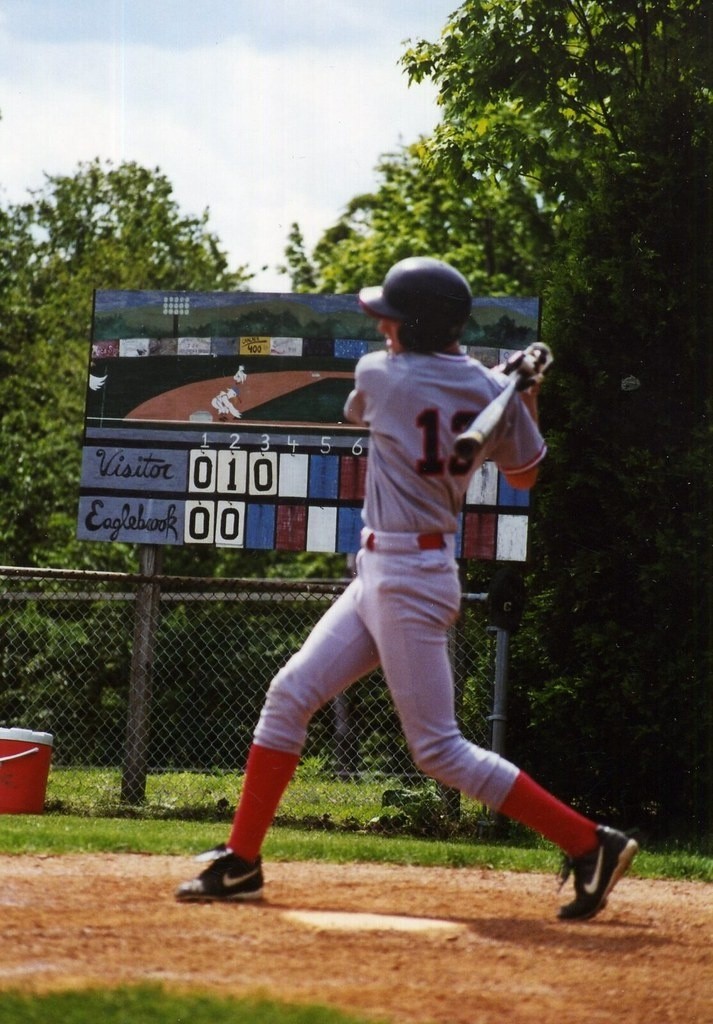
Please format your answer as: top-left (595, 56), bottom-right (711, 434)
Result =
top-left (554, 825), bottom-right (638, 922)
top-left (173, 844), bottom-right (264, 903)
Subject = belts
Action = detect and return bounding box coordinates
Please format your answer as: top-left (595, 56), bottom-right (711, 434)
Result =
top-left (367, 533), bottom-right (448, 550)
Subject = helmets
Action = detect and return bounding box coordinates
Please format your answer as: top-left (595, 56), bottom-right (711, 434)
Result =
top-left (359, 256), bottom-right (473, 354)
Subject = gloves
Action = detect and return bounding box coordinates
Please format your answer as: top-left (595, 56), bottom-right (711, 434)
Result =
top-left (492, 342), bottom-right (554, 390)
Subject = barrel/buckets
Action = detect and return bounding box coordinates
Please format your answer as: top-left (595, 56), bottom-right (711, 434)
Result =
top-left (0, 727), bottom-right (53, 814)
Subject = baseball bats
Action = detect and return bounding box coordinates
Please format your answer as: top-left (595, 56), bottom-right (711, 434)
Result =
top-left (453, 363), bottom-right (532, 447)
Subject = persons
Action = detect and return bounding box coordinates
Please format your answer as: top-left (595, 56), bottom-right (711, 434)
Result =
top-left (175, 255), bottom-right (640, 923)
